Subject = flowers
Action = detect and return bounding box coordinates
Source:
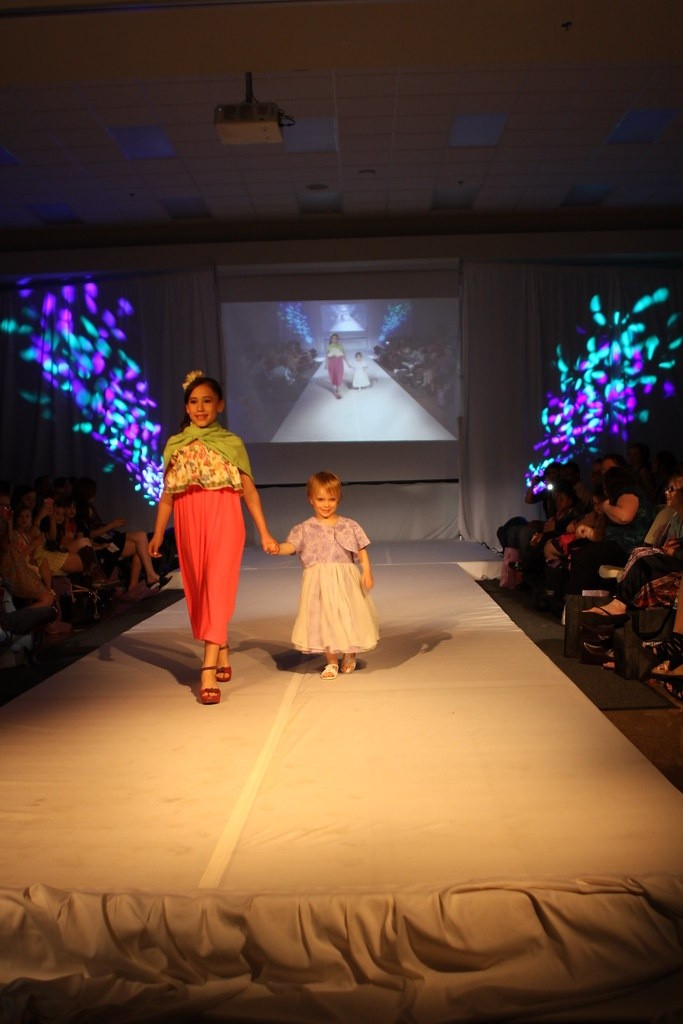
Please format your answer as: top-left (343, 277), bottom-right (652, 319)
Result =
top-left (181, 369), bottom-right (206, 393)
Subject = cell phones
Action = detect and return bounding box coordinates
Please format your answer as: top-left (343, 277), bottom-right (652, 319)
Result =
top-left (534, 475), bottom-right (546, 481)
top-left (43, 498), bottom-right (54, 515)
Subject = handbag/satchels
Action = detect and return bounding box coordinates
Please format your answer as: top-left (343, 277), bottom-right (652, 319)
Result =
top-left (499, 547), bottom-right (524, 588)
top-left (60, 585), bottom-right (99, 625)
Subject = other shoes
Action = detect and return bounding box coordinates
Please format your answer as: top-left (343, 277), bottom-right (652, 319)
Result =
top-left (579, 607), bottom-right (628, 623)
top-left (602, 661), bottom-right (616, 670)
top-left (651, 659), bottom-right (683, 676)
top-left (508, 562), bottom-right (540, 573)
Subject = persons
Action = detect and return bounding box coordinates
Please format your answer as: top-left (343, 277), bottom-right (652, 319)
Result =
top-left (374, 333), bottom-right (457, 409)
top-left (147, 370), bottom-right (279, 705)
top-left (495, 453), bottom-right (683, 698)
top-left (350, 351), bottom-right (370, 390)
top-left (325, 333), bottom-right (349, 399)
top-left (267, 471), bottom-right (380, 679)
top-left (0, 480), bottom-right (173, 672)
top-left (226, 341), bottom-right (317, 422)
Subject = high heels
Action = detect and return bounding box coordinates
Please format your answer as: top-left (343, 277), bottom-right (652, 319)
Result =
top-left (214, 643), bottom-right (232, 683)
top-left (199, 665), bottom-right (221, 705)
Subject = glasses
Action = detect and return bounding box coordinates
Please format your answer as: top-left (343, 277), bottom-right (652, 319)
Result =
top-left (664, 487), bottom-right (683, 492)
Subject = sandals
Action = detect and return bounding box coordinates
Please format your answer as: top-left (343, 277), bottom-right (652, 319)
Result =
top-left (340, 651), bottom-right (357, 673)
top-left (642, 633), bottom-right (683, 660)
top-left (321, 663), bottom-right (338, 679)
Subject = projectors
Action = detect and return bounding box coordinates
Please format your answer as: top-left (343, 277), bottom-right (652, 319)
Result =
top-left (215, 102), bottom-right (284, 144)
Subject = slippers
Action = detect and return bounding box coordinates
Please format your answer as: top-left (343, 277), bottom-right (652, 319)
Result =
top-left (148, 575), bottom-right (173, 593)
